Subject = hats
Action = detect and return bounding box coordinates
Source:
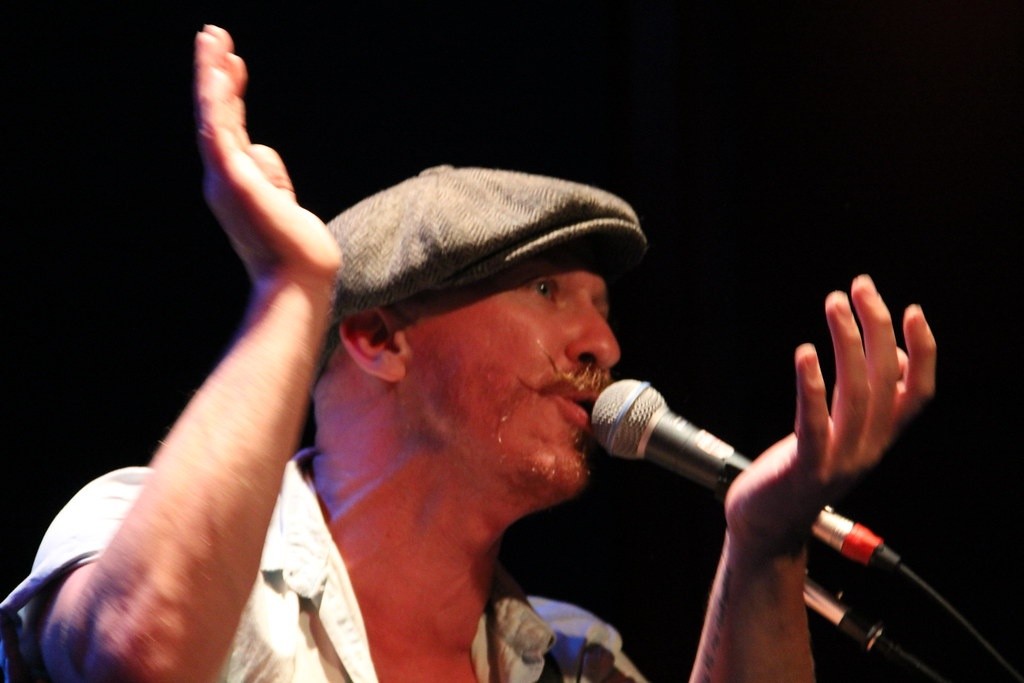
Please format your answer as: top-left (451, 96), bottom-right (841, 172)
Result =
top-left (323, 165), bottom-right (648, 328)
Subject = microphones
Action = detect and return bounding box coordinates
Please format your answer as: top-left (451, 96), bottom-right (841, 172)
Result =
top-left (591, 380), bottom-right (901, 572)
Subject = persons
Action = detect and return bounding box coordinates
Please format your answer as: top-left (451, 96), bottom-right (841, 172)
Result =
top-left (0, 23), bottom-right (937, 683)
